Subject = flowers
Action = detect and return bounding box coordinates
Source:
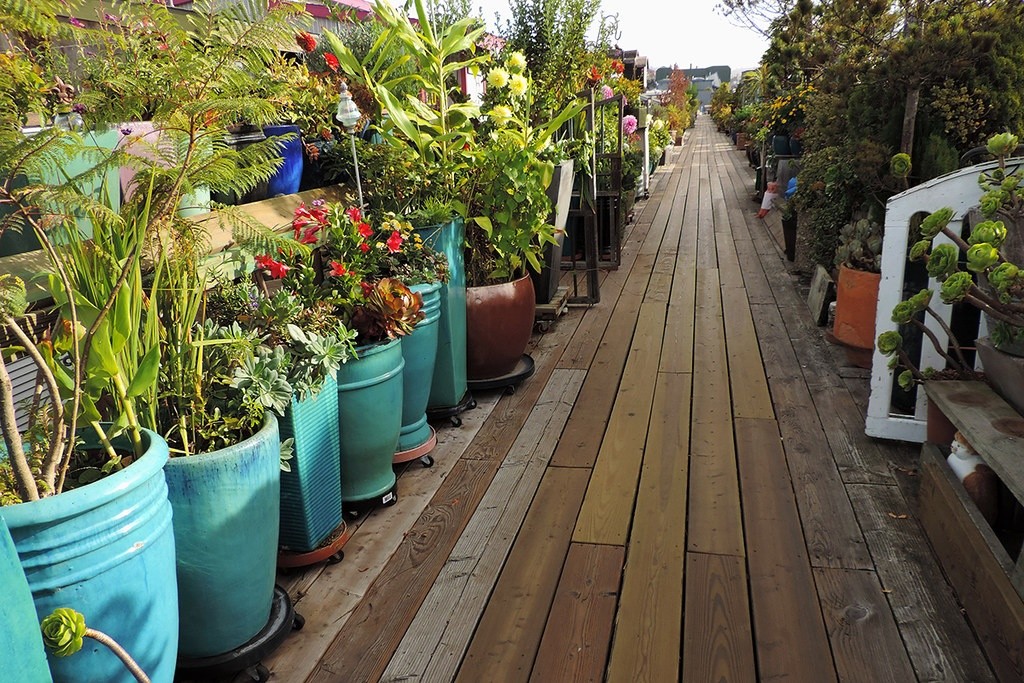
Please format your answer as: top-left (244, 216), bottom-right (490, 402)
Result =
top-left (483, 47), bottom-right (585, 162)
top-left (768, 84), bottom-right (818, 136)
top-left (586, 56), bottom-right (674, 157)
top-left (248, 196), bottom-right (426, 335)
top-left (292, 200), bottom-right (451, 286)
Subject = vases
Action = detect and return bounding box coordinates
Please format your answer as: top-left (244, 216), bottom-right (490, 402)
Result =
top-left (623, 131), bottom-right (678, 211)
top-left (337, 335), bottom-right (406, 503)
top-left (303, 135), bottom-right (342, 188)
top-left (790, 137), bottom-right (802, 156)
top-left (528, 160), bottom-right (577, 305)
top-left (391, 278), bottom-right (442, 455)
top-left (772, 135), bottom-right (791, 155)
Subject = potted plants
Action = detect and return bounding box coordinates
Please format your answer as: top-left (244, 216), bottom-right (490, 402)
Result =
top-left (781, 131), bottom-right (1024, 413)
top-left (0, 0), bottom-right (588, 683)
